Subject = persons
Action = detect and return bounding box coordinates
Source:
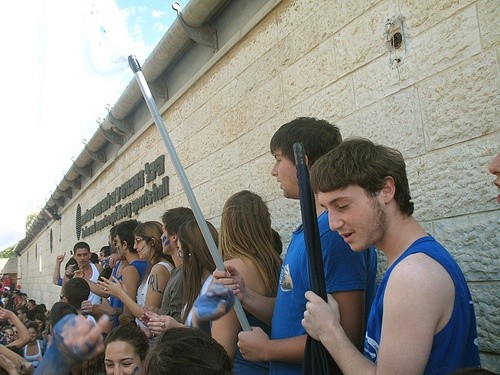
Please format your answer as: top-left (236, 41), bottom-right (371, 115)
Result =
top-left (0, 116), bottom-right (500, 375)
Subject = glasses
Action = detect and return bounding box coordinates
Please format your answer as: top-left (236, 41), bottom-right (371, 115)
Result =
top-left (134, 238), bottom-right (148, 244)
top-left (59, 294), bottom-right (65, 300)
top-left (113, 239), bottom-right (126, 247)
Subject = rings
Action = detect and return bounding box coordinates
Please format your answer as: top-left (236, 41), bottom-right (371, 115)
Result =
top-left (104, 288), bottom-right (108, 292)
top-left (161, 323), bottom-right (165, 329)
